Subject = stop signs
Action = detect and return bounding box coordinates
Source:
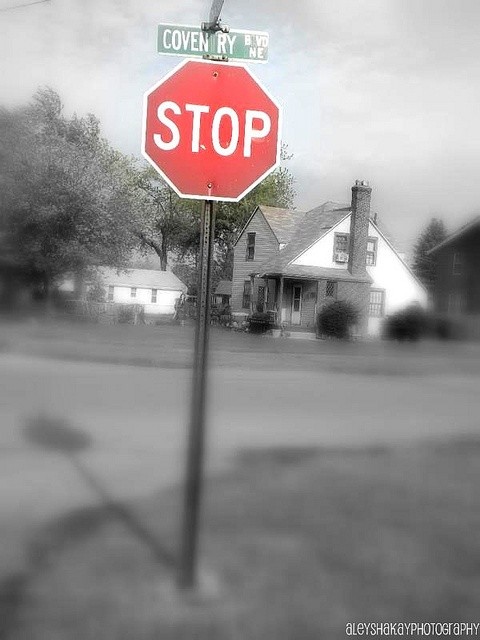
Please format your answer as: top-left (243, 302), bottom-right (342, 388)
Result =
top-left (140, 57), bottom-right (281, 206)
